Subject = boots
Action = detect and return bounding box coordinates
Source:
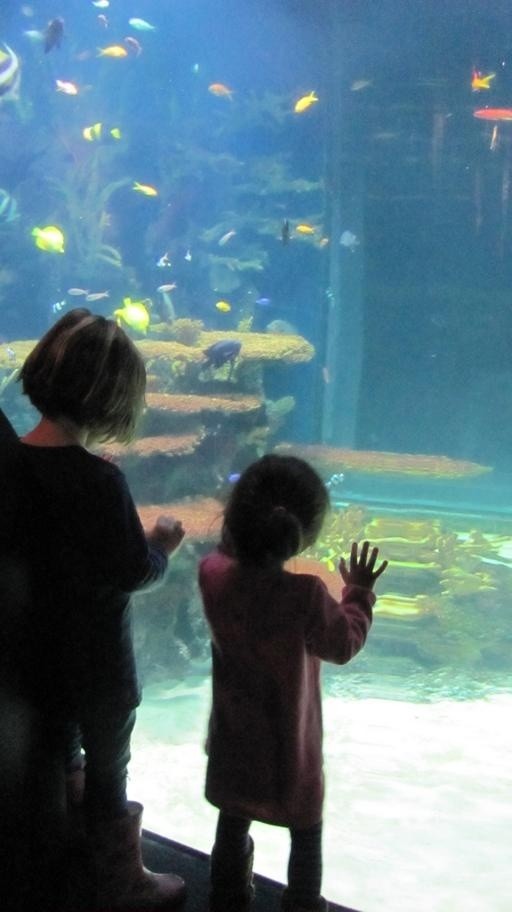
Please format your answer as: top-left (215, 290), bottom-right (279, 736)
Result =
top-left (206, 835), bottom-right (258, 912)
top-left (74, 800), bottom-right (187, 908)
top-left (42, 754), bottom-right (88, 816)
top-left (278, 886), bottom-right (329, 912)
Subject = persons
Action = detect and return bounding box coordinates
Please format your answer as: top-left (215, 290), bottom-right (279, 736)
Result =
top-left (1, 307), bottom-right (188, 906)
top-left (198, 453), bottom-right (388, 912)
